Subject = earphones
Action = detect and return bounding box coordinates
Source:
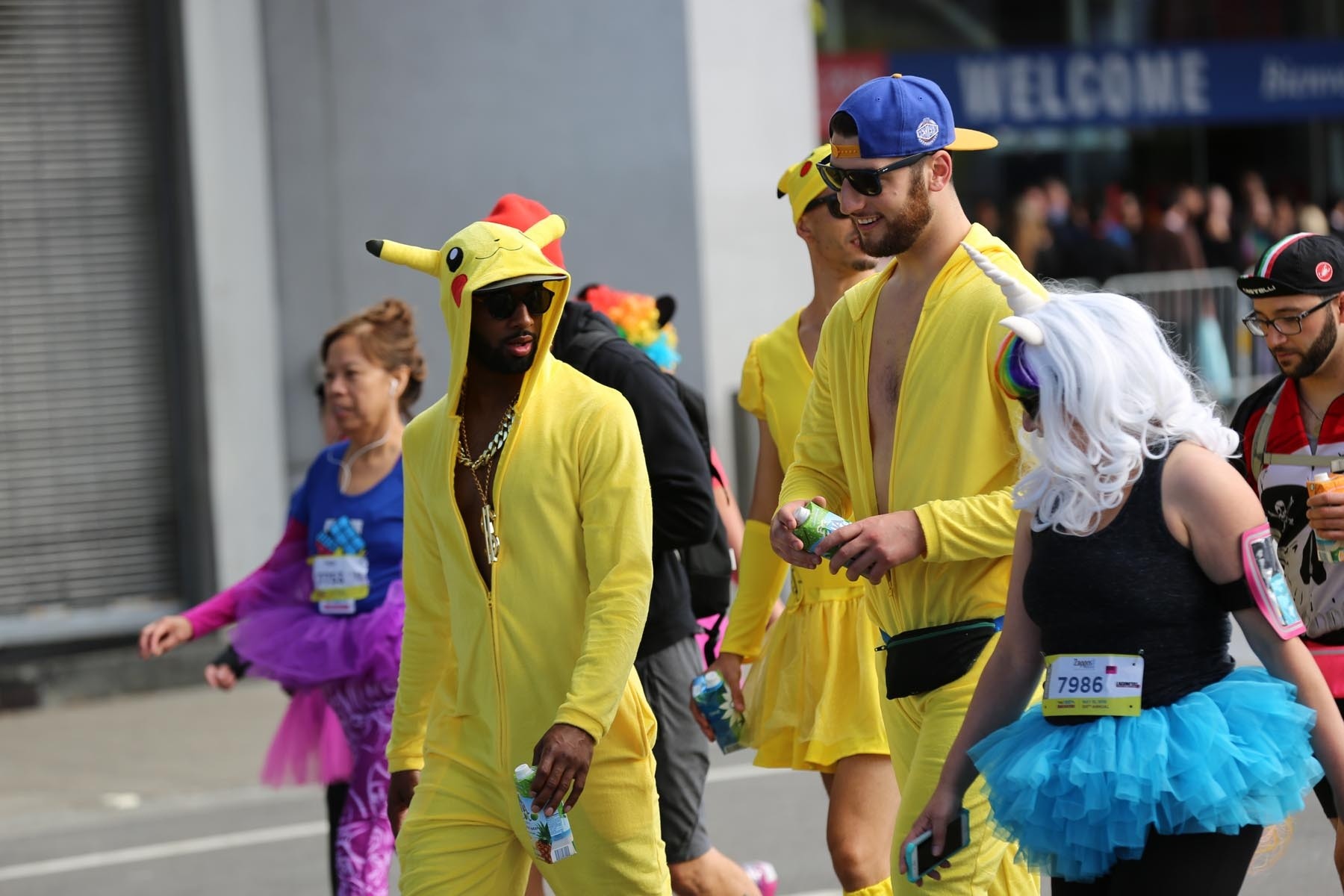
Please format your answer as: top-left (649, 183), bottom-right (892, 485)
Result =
top-left (390, 377), bottom-right (398, 396)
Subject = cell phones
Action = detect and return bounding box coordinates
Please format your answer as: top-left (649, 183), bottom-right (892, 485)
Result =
top-left (905, 807), bottom-right (971, 883)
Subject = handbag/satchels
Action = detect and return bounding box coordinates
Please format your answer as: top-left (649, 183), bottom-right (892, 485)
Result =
top-left (886, 618), bottom-right (998, 700)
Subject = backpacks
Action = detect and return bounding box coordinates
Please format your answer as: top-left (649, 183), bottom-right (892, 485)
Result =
top-left (565, 332), bottom-right (730, 618)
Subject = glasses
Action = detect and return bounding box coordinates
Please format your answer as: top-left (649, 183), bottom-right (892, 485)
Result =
top-left (816, 149), bottom-right (938, 196)
top-left (1017, 393), bottom-right (1039, 422)
top-left (803, 192), bottom-right (850, 219)
top-left (472, 285), bottom-right (555, 320)
top-left (1242, 295), bottom-right (1338, 337)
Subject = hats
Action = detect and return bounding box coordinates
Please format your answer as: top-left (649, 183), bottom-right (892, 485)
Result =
top-left (1237, 232), bottom-right (1344, 299)
top-left (829, 74), bottom-right (998, 159)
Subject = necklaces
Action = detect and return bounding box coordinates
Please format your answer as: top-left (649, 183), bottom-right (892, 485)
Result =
top-left (456, 396), bottom-right (518, 564)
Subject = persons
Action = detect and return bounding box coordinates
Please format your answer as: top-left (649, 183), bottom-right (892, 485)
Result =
top-left (365, 215), bottom-right (673, 896)
top-left (483, 74), bottom-right (1344, 895)
top-left (141, 301), bottom-right (426, 896)
